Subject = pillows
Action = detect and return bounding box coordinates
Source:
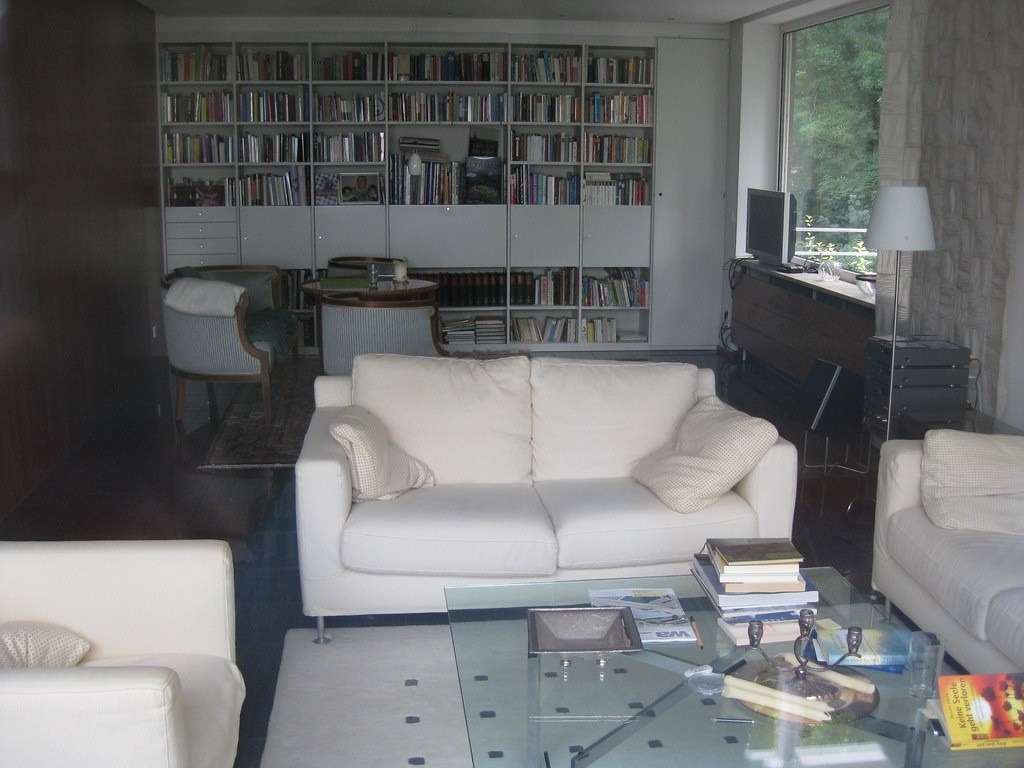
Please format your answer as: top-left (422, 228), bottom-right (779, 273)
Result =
top-left (921, 429), bottom-right (1024, 535)
top-left (0, 621), bottom-right (90, 669)
top-left (630, 395), bottom-right (778, 514)
top-left (330, 405), bottom-right (436, 503)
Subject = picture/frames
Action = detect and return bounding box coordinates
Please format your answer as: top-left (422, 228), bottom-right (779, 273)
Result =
top-left (339, 172), bottom-right (380, 205)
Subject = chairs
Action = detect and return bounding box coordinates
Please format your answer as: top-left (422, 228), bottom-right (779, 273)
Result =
top-left (321, 289), bottom-right (445, 375)
top-left (327, 256), bottom-right (402, 301)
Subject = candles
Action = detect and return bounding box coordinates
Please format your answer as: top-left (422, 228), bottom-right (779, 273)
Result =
top-left (391, 256), bottom-right (409, 282)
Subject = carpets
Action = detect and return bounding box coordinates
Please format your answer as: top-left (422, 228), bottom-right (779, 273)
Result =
top-left (230, 481), bottom-right (1024, 768)
top-left (194, 354), bottom-right (324, 467)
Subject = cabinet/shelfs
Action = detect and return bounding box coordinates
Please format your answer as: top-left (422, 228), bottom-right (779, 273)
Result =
top-left (859, 333), bottom-right (971, 440)
top-left (155, 31), bottom-right (660, 351)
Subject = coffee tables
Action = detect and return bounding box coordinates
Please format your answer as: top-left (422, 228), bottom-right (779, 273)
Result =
top-left (443, 567), bottom-right (1024, 768)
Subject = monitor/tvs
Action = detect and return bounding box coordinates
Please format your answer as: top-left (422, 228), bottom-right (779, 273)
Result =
top-left (745, 188), bottom-right (797, 265)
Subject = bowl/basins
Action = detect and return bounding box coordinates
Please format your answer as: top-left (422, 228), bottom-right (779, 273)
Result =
top-left (856, 275), bottom-right (876, 300)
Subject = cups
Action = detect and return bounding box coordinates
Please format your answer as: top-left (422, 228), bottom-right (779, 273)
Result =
top-left (368, 264), bottom-right (379, 289)
top-left (908, 631), bottom-right (945, 700)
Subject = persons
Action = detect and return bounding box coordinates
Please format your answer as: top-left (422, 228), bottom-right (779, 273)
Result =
top-left (342, 176), bottom-right (378, 201)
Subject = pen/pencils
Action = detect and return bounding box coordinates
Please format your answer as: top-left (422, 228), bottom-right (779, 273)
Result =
top-left (722, 659), bottom-right (746, 675)
top-left (710, 717), bottom-right (755, 724)
top-left (689, 614), bottom-right (703, 649)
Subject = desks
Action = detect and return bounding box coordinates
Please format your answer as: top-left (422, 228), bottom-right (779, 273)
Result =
top-left (298, 275), bottom-right (440, 301)
top-left (728, 254), bottom-right (876, 432)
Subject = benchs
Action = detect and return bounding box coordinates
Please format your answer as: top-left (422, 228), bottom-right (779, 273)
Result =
top-left (160, 264), bottom-right (295, 432)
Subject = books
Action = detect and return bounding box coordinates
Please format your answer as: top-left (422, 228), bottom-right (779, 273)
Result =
top-left (315, 172), bottom-right (339, 206)
top-left (690, 539), bottom-right (818, 646)
top-left (510, 50), bottom-right (581, 206)
top-left (915, 673), bottom-right (1024, 751)
top-left (312, 51), bottom-right (386, 162)
top-left (583, 53), bottom-right (655, 205)
top-left (437, 315), bottom-right (506, 345)
top-left (380, 177), bottom-right (385, 205)
top-left (582, 268), bottom-right (648, 308)
top-left (388, 90), bottom-right (508, 121)
top-left (237, 51), bottom-right (311, 206)
top-left (279, 269), bottom-right (330, 346)
top-left (588, 586), bottom-right (698, 645)
top-left (408, 272), bottom-right (506, 306)
top-left (159, 45), bottom-right (235, 207)
top-left (510, 268), bottom-right (579, 306)
top-left (803, 629), bottom-right (928, 674)
top-left (389, 124), bottom-right (507, 205)
top-left (388, 51), bottom-right (508, 81)
top-left (509, 313), bottom-right (578, 342)
top-left (581, 317), bottom-right (647, 344)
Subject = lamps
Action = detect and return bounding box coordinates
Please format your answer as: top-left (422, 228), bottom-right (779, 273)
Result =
top-left (853, 185), bottom-right (938, 512)
top-left (408, 149), bottom-right (422, 177)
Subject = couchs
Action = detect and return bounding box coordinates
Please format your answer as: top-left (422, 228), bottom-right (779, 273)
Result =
top-left (869, 428), bottom-right (1024, 674)
top-left (292, 353), bottom-right (799, 643)
top-left (0, 539), bottom-right (245, 768)
